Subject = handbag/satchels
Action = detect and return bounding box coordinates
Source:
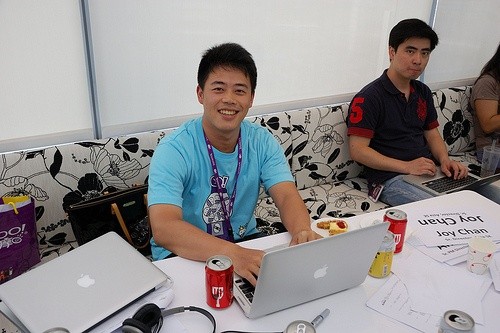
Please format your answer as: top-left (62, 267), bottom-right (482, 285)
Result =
top-left (68, 183), bottom-right (152, 257)
top-left (0, 196), bottom-right (41, 285)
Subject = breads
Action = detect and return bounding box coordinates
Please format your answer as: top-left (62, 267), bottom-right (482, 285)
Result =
top-left (317, 220), bottom-right (348, 236)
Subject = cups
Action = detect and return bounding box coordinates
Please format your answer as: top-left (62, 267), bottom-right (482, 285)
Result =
top-left (480, 146), bottom-right (500, 177)
top-left (467, 236), bottom-right (497, 274)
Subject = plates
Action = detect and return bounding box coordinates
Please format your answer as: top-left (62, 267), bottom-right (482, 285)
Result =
top-left (311, 217), bottom-right (361, 238)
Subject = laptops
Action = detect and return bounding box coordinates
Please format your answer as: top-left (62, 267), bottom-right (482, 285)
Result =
top-left (232, 222), bottom-right (391, 321)
top-left (402, 171), bottom-right (500, 196)
top-left (1, 229), bottom-right (168, 333)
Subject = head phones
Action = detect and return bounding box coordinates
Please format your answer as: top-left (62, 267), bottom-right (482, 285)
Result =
top-left (123, 303), bottom-right (217, 332)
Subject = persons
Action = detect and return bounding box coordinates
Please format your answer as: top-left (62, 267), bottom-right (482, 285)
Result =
top-left (470, 43), bottom-right (500, 168)
top-left (345, 19), bottom-right (469, 206)
top-left (148, 43), bottom-right (325, 288)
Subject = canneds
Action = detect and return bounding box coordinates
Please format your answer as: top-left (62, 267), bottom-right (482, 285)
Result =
top-left (438, 309), bottom-right (475, 333)
top-left (369, 232), bottom-right (396, 278)
top-left (285, 319), bottom-right (317, 333)
top-left (383, 209), bottom-right (407, 253)
top-left (205, 255), bottom-right (235, 308)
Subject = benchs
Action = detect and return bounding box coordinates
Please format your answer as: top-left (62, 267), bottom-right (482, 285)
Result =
top-left (0, 85), bottom-right (479, 270)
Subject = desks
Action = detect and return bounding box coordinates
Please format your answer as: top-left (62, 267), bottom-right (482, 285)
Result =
top-left (115, 190), bottom-right (500, 333)
